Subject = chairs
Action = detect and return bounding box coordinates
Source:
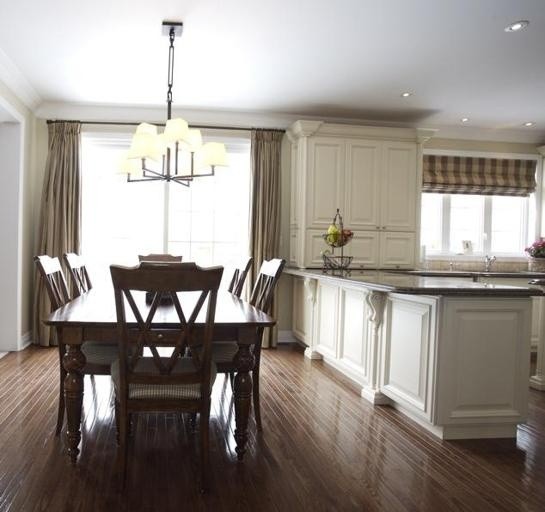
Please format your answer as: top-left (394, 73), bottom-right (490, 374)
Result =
top-left (34, 252), bottom-right (142, 443)
top-left (110, 254), bottom-right (224, 494)
top-left (185, 255), bottom-right (284, 429)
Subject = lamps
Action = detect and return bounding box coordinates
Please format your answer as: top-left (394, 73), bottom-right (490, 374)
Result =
top-left (120, 18), bottom-right (228, 185)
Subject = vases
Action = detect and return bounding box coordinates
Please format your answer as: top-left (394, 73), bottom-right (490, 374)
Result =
top-left (528, 258), bottom-right (545, 272)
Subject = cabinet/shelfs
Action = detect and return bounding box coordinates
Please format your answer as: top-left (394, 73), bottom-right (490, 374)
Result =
top-left (344, 136), bottom-right (422, 272)
top-left (289, 135), bottom-right (344, 271)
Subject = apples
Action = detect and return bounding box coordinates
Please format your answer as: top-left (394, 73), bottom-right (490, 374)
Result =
top-left (326, 225), bottom-right (352, 245)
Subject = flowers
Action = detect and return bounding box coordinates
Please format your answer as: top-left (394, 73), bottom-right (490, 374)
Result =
top-left (525, 239), bottom-right (545, 259)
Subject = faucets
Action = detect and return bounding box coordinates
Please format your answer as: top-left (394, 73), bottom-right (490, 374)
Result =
top-left (481, 255), bottom-right (497, 271)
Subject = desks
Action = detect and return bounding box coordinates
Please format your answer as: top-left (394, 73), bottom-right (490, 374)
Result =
top-left (38, 280), bottom-right (277, 467)
